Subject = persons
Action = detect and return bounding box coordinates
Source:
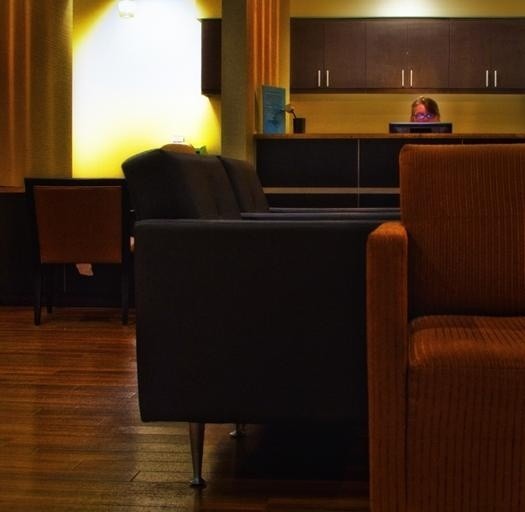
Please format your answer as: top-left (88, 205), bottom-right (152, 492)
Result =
top-left (409, 97), bottom-right (440, 124)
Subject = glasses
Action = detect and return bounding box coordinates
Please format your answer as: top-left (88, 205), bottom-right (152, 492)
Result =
top-left (412, 113), bottom-right (437, 119)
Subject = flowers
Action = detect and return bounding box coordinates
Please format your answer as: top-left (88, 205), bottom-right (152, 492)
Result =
top-left (284, 104), bottom-right (297, 118)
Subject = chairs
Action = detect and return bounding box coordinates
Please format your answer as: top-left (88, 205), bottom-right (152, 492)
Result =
top-left (218, 154), bottom-right (400, 221)
top-left (22, 177), bottom-right (133, 327)
top-left (365, 143), bottom-right (524, 512)
top-left (119, 148), bottom-right (401, 489)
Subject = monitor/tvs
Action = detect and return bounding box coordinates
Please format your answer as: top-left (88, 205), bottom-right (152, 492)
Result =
top-left (389, 122), bottom-right (452, 134)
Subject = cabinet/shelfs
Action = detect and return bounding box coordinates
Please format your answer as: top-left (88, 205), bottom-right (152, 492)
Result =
top-left (449, 17), bottom-right (525, 93)
top-left (364, 17), bottom-right (449, 93)
top-left (289, 17), bottom-right (367, 93)
